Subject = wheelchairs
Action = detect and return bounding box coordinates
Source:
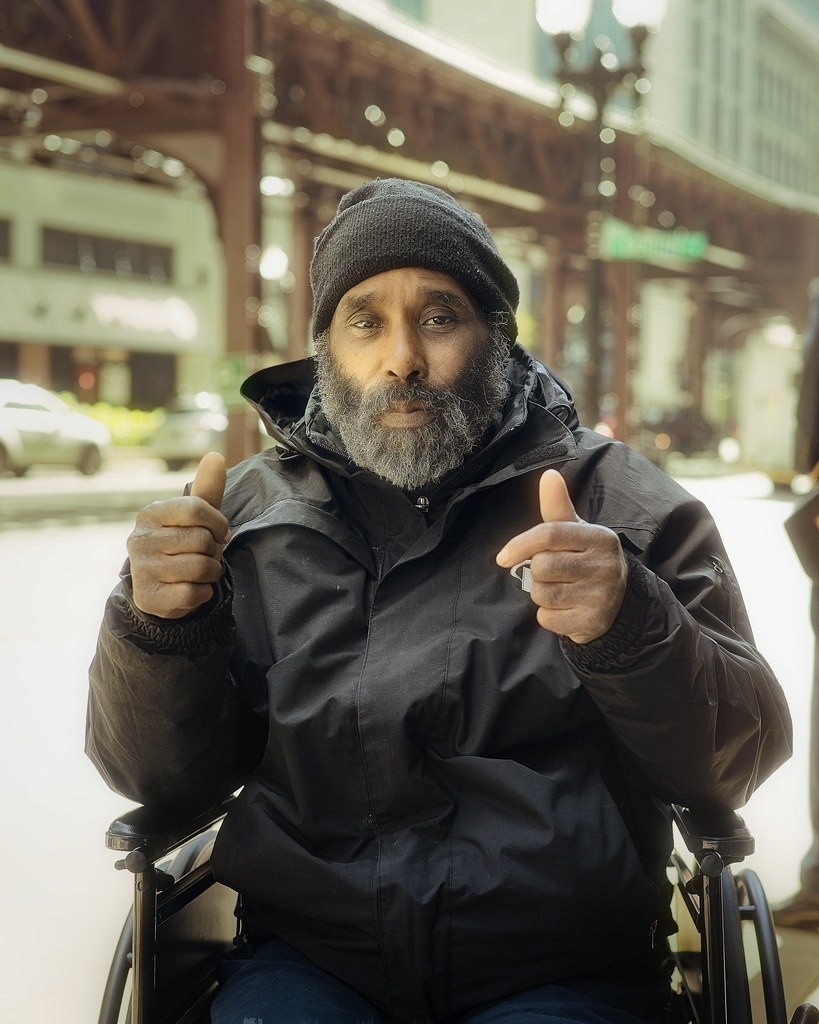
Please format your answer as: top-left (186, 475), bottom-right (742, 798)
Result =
top-left (96, 778), bottom-right (790, 1023)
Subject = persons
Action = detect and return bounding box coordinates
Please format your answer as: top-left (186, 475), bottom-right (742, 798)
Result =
top-left (766, 286), bottom-right (819, 933)
top-left (85, 174), bottom-right (792, 1024)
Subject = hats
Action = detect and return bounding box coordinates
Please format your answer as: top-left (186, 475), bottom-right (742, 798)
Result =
top-left (309, 177), bottom-right (526, 348)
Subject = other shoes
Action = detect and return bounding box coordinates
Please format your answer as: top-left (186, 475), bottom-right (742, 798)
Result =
top-left (766, 891), bottom-right (819, 929)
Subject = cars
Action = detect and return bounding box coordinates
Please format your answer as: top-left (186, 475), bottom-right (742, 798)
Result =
top-left (0, 379), bottom-right (112, 478)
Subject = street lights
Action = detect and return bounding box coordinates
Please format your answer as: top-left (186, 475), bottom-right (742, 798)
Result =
top-left (536, 0), bottom-right (668, 422)
